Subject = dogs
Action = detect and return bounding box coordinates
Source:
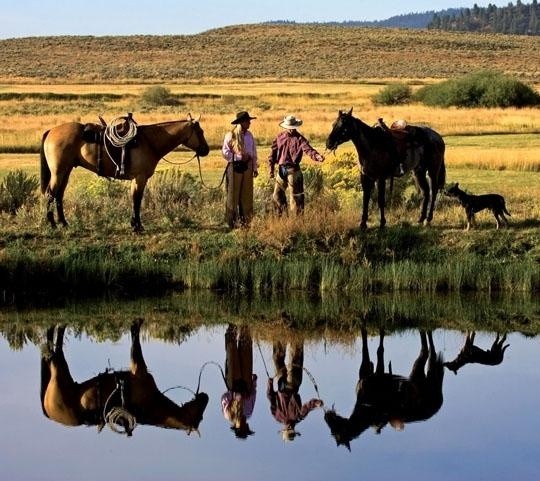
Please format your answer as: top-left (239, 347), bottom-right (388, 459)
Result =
top-left (443, 331), bottom-right (510, 375)
top-left (443, 182), bottom-right (509, 234)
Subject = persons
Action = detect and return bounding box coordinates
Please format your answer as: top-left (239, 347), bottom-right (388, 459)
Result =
top-left (266, 340), bottom-right (324, 441)
top-left (220, 323), bottom-right (257, 439)
top-left (221, 111), bottom-right (260, 230)
top-left (267, 115), bottom-right (326, 217)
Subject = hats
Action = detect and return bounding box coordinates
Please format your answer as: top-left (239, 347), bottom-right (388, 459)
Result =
top-left (280, 431), bottom-right (301, 441)
top-left (230, 427), bottom-right (255, 438)
top-left (279, 116), bottom-right (303, 129)
top-left (231, 112), bottom-right (257, 124)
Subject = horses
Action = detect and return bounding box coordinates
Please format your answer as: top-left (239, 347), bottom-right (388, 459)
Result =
top-left (40, 316), bottom-right (209, 440)
top-left (324, 106), bottom-right (448, 231)
top-left (322, 324), bottom-right (444, 452)
top-left (36, 114), bottom-right (212, 233)
